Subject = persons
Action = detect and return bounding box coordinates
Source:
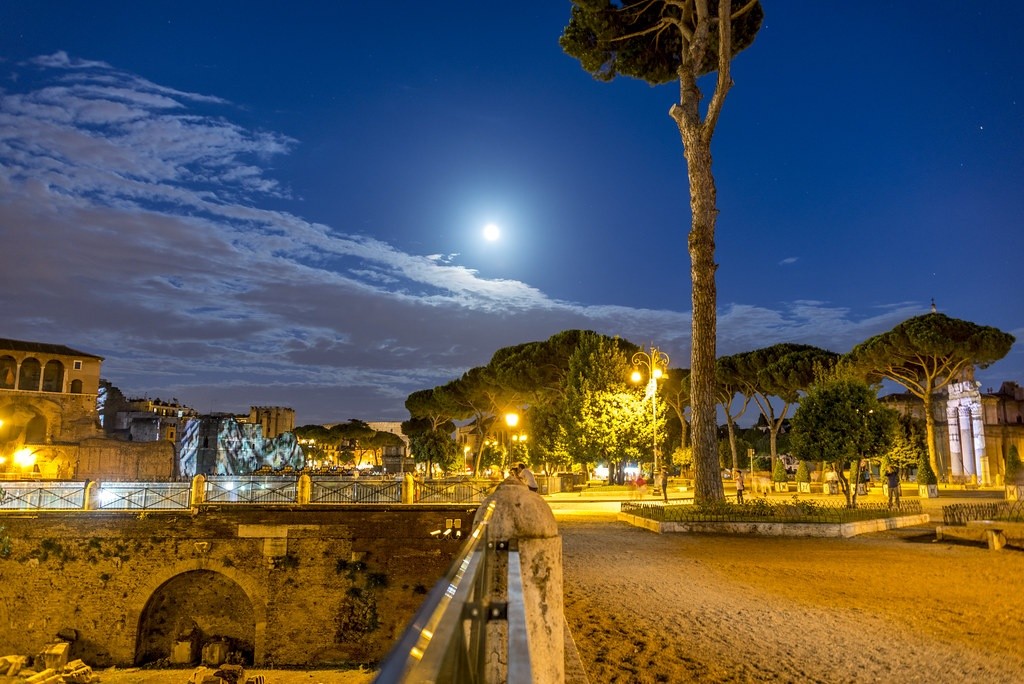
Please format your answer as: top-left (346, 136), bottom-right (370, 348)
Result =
top-left (661, 466), bottom-right (669, 504)
top-left (734, 470), bottom-right (744, 504)
top-left (860, 469), bottom-right (870, 491)
top-left (884, 465), bottom-right (900, 510)
top-left (508, 463), bottom-right (538, 491)
top-left (636, 476), bottom-right (644, 498)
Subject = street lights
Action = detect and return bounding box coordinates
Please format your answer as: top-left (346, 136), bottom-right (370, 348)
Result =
top-left (631, 347), bottom-right (669, 496)
top-left (506, 409), bottom-right (518, 469)
top-left (460, 443), bottom-right (470, 475)
top-left (511, 428), bottom-right (527, 463)
top-left (485, 436), bottom-right (498, 466)
